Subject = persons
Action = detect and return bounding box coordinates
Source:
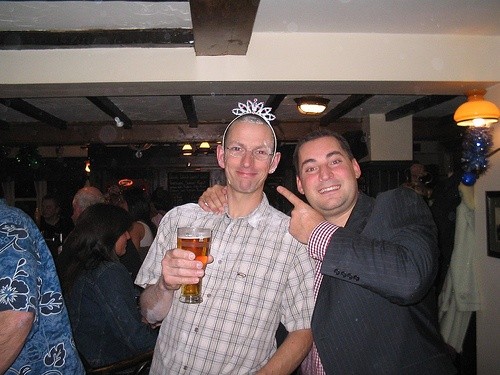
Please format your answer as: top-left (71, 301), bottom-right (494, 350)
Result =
top-left (199, 129), bottom-right (474, 375)
top-left (0, 184), bottom-right (167, 375)
top-left (133, 97), bottom-right (315, 375)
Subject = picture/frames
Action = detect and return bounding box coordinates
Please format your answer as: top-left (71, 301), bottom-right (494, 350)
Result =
top-left (484, 190), bottom-right (500, 259)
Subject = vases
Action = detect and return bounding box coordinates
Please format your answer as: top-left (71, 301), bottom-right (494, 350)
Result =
top-left (474, 153), bottom-right (486, 169)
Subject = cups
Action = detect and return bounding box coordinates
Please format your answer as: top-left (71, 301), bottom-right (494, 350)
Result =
top-left (178, 227), bottom-right (212, 304)
top-left (53, 232), bottom-right (62, 244)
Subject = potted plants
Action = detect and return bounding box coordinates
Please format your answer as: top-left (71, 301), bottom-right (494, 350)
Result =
top-left (457, 147), bottom-right (479, 185)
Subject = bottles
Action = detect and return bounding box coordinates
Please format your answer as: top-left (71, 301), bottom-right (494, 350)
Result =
top-left (118, 185), bottom-right (126, 203)
top-left (34, 207), bottom-right (41, 223)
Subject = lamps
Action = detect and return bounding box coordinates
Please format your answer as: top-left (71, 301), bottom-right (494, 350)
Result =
top-left (454, 88), bottom-right (500, 127)
top-left (293, 96), bottom-right (331, 117)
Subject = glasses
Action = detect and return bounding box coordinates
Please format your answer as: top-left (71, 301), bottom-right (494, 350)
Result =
top-left (224, 145), bottom-right (274, 160)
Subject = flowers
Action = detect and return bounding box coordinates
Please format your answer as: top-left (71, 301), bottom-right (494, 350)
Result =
top-left (460, 125), bottom-right (494, 149)
